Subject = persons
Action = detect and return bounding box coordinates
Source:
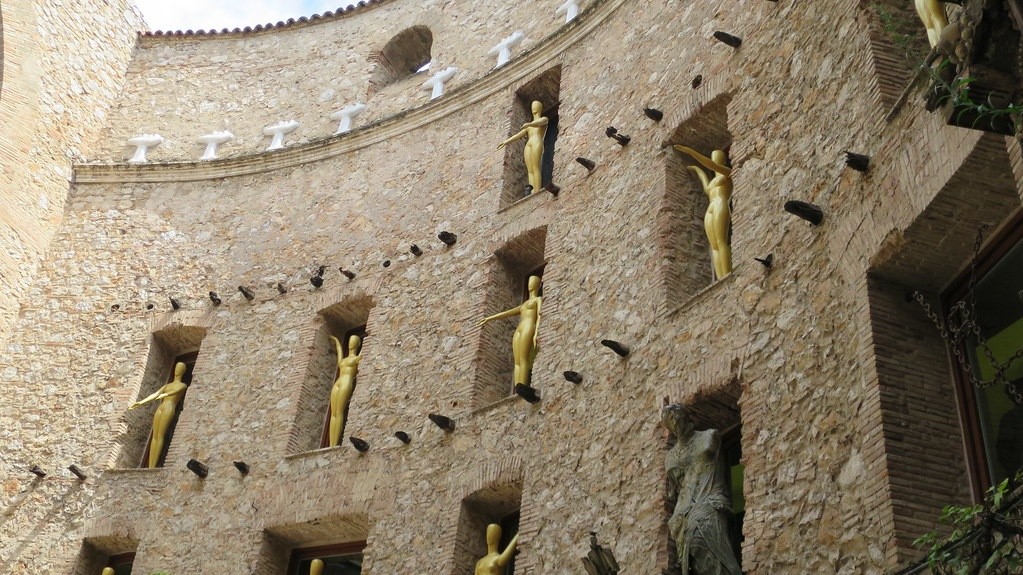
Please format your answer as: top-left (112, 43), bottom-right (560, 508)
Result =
top-left (475, 524), bottom-right (519, 575)
top-left (476, 275), bottom-right (542, 386)
top-left (129, 362), bottom-right (187, 468)
top-left (915, 0), bottom-right (949, 49)
top-left (310, 559), bottom-right (324, 575)
top-left (660, 404), bottom-right (742, 575)
top-left (674, 144), bottom-right (732, 279)
top-left (496, 101), bottom-right (548, 194)
top-left (329, 335), bottom-right (362, 447)
